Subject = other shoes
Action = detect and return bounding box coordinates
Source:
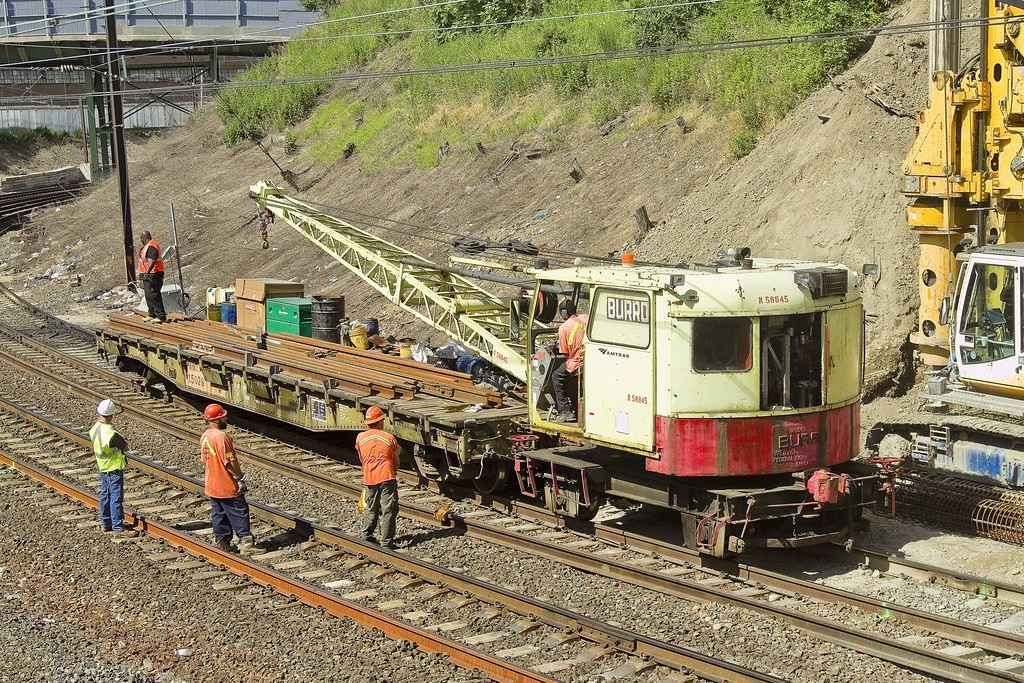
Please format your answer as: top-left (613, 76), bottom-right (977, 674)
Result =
top-left (150, 318), bottom-right (165, 324)
top-left (101, 527), bottom-right (113, 532)
top-left (141, 315), bottom-right (155, 321)
top-left (551, 410), bottom-right (574, 422)
top-left (113, 530), bottom-right (138, 538)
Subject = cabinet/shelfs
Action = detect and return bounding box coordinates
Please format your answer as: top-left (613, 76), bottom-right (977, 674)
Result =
top-left (207, 277), bottom-right (313, 337)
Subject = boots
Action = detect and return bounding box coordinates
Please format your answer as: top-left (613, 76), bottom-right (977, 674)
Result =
top-left (214, 540), bottom-right (238, 554)
top-left (240, 535), bottom-right (266, 556)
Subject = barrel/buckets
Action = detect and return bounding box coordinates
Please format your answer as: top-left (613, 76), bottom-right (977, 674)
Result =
top-left (310, 295), bottom-right (345, 344)
top-left (208, 304), bottom-right (222, 322)
top-left (361, 318), bottom-right (378, 337)
top-left (221, 302), bottom-right (237, 324)
top-left (348, 326), bottom-right (369, 351)
top-left (399, 338), bottom-right (416, 358)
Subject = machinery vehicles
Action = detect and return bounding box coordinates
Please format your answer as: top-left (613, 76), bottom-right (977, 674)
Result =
top-left (95, 180), bottom-right (882, 559)
top-left (867, 245), bottom-right (1024, 544)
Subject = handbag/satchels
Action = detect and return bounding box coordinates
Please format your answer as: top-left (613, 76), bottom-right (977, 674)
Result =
top-left (357, 486), bottom-right (367, 514)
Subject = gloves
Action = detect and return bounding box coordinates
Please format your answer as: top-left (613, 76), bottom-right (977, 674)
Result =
top-left (143, 273), bottom-right (152, 282)
top-left (236, 476), bottom-right (248, 493)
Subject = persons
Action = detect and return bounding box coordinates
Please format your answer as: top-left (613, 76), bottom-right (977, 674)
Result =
top-left (89, 399), bottom-right (138, 538)
top-left (138, 231), bottom-right (167, 323)
top-left (199, 404), bottom-right (266, 556)
top-left (355, 407), bottom-right (401, 550)
top-left (552, 300), bottom-right (590, 423)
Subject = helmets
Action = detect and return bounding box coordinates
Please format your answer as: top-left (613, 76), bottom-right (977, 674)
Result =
top-left (97, 399), bottom-right (115, 416)
top-left (364, 407), bottom-right (386, 424)
top-left (204, 403), bottom-right (227, 419)
